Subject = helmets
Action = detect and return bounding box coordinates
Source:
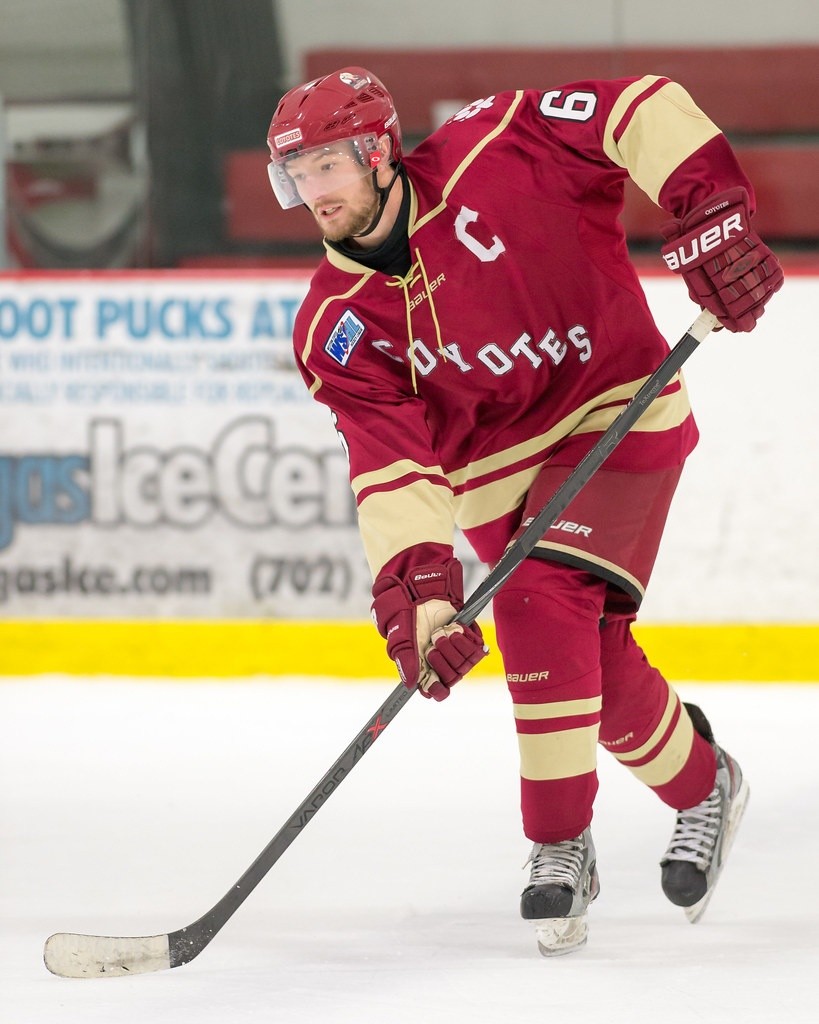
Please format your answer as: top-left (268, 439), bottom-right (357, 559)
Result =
top-left (267, 65), bottom-right (403, 209)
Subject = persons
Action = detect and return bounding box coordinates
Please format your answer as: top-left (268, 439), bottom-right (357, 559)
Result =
top-left (269, 66), bottom-right (784, 958)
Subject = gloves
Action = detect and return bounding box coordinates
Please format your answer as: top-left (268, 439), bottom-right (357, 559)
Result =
top-left (371, 558), bottom-right (490, 703)
top-left (660, 187), bottom-right (784, 334)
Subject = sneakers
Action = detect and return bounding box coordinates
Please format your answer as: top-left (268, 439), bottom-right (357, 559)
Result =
top-left (660, 703), bottom-right (750, 922)
top-left (520, 823), bottom-right (601, 957)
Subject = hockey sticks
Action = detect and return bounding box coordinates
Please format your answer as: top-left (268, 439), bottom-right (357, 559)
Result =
top-left (39, 306), bottom-right (722, 979)
